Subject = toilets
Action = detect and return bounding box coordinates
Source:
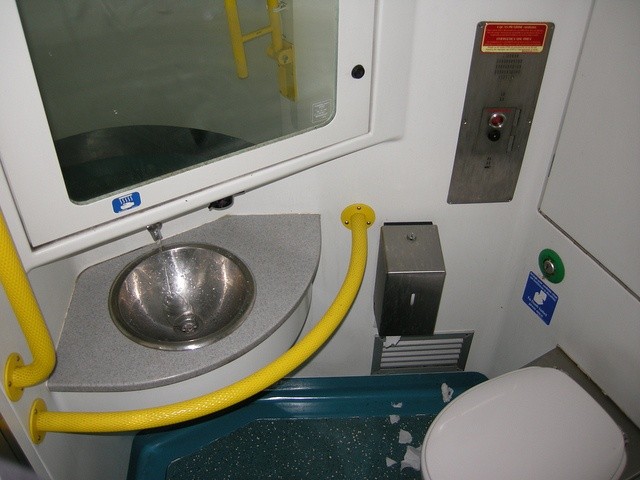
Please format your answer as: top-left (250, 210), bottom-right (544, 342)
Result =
top-left (420, 347), bottom-right (640, 480)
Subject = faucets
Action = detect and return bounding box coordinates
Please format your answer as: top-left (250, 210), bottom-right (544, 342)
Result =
top-left (145, 224), bottom-right (164, 242)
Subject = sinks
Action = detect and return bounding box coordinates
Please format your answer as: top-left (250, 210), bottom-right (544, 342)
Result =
top-left (108, 241), bottom-right (256, 351)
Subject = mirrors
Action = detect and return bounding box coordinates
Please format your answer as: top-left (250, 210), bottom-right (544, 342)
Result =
top-left (15, 0), bottom-right (339, 205)
